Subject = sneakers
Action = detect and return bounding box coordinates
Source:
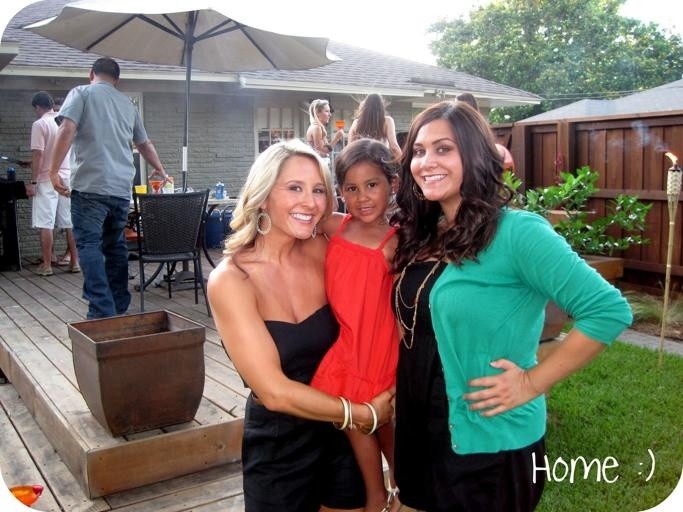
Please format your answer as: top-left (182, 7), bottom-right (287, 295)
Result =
top-left (32, 262), bottom-right (53, 276)
top-left (57, 254), bottom-right (71, 265)
top-left (68, 260), bottom-right (81, 273)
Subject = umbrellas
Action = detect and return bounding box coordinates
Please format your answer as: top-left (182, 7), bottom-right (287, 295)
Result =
top-left (21, 1), bottom-right (344, 196)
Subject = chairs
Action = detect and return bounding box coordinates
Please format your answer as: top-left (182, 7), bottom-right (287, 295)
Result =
top-left (127, 189), bottom-right (212, 313)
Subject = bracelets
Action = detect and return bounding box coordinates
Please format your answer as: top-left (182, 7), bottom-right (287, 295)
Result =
top-left (332, 395), bottom-right (348, 431)
top-left (344, 397), bottom-right (353, 432)
top-left (354, 401), bottom-right (377, 437)
top-left (28, 179), bottom-right (38, 186)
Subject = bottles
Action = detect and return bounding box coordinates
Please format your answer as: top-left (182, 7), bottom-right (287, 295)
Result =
top-left (215, 181), bottom-right (224, 199)
top-left (160, 176), bottom-right (175, 195)
top-left (6, 166), bottom-right (15, 183)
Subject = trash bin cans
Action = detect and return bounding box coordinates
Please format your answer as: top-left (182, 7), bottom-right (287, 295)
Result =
top-left (67, 309), bottom-right (206, 438)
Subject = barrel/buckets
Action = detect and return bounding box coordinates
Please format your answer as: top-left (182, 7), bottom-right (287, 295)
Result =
top-left (205, 209), bottom-right (234, 247)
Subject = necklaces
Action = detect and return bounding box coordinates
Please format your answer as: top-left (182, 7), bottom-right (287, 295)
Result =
top-left (392, 230), bottom-right (443, 351)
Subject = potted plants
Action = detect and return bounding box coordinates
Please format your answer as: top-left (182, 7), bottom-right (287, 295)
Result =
top-left (539, 167), bottom-right (654, 342)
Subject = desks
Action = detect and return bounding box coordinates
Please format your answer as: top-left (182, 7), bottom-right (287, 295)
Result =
top-left (208, 198), bottom-right (237, 232)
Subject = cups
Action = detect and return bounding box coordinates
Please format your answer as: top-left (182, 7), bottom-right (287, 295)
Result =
top-left (148, 180), bottom-right (164, 193)
top-left (335, 120), bottom-right (344, 129)
top-left (134, 184), bottom-right (147, 194)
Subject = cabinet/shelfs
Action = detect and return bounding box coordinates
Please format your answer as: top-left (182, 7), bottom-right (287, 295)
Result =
top-left (1, 179), bottom-right (31, 272)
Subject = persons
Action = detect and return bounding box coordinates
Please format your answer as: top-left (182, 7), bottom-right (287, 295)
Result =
top-left (302, 99), bottom-right (344, 160)
top-left (312, 143), bottom-right (414, 512)
top-left (347, 92), bottom-right (402, 165)
top-left (46, 56), bottom-right (175, 324)
top-left (382, 98), bottom-right (637, 510)
top-left (23, 90), bottom-right (82, 277)
top-left (204, 136), bottom-right (404, 511)
top-left (455, 92), bottom-right (478, 115)
top-left (44, 97), bottom-right (77, 267)
